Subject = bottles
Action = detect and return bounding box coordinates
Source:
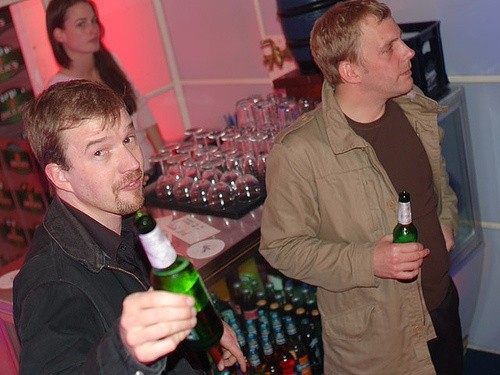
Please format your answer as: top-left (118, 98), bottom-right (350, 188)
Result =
top-left (392, 192), bottom-right (420, 284)
top-left (134, 209), bottom-right (224, 349)
top-left (212, 267), bottom-right (325, 375)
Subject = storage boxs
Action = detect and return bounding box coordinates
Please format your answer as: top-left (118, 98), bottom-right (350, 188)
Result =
top-left (398, 21), bottom-right (451, 102)
top-left (437, 86), bottom-right (484, 270)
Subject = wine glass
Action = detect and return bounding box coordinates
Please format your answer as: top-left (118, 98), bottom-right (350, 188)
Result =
top-left (151, 126), bottom-right (279, 209)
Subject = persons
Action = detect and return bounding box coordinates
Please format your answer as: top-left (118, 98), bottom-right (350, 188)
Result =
top-left (258, 0), bottom-right (464, 375)
top-left (12, 80), bottom-right (247, 375)
top-left (46, 0), bottom-right (166, 190)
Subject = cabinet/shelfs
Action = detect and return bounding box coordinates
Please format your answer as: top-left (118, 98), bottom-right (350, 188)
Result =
top-left (0, 0), bottom-right (264, 372)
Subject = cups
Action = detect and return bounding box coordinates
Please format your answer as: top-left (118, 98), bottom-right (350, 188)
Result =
top-left (236, 95), bottom-right (315, 131)
